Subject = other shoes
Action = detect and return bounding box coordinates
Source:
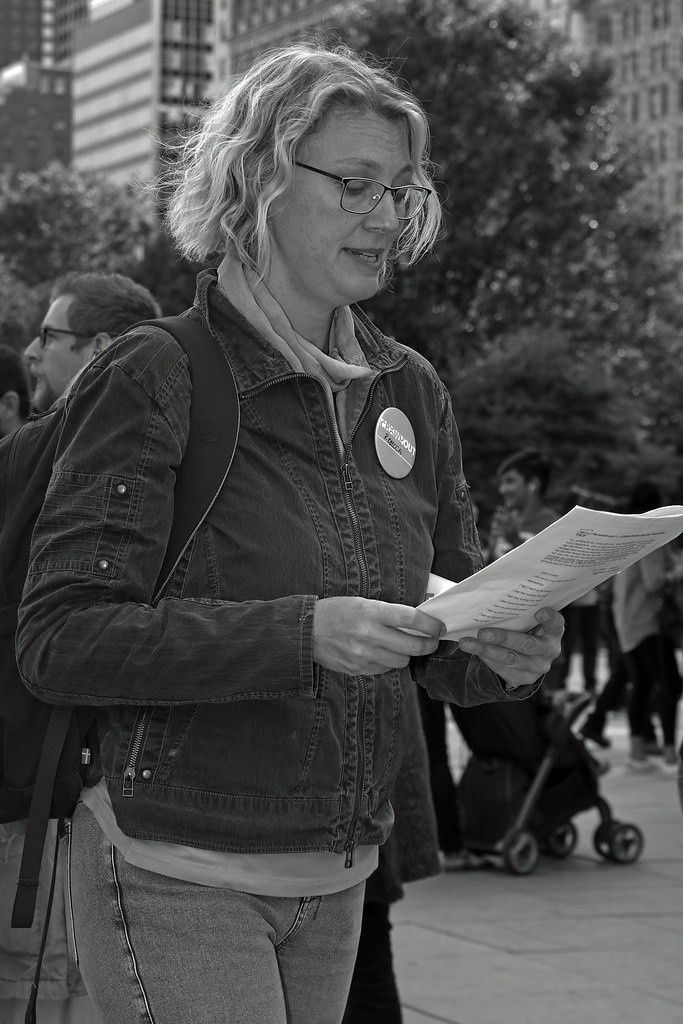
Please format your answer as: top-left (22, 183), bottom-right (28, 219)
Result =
top-left (624, 757), bottom-right (652, 771)
top-left (579, 727), bottom-right (610, 748)
top-left (587, 751), bottom-right (609, 776)
top-left (646, 755), bottom-right (680, 776)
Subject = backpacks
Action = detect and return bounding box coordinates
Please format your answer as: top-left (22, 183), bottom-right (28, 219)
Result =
top-left (1, 315), bottom-right (242, 825)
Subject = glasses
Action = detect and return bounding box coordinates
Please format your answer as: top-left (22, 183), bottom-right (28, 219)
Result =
top-left (39, 324), bottom-right (120, 349)
top-left (291, 159), bottom-right (432, 221)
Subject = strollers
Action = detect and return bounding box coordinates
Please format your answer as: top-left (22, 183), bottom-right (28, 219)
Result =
top-left (450, 684), bottom-right (644, 874)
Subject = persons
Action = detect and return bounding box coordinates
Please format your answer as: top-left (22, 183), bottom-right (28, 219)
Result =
top-left (16, 45), bottom-right (565, 1024)
top-left (0, 273), bottom-right (683, 1024)
